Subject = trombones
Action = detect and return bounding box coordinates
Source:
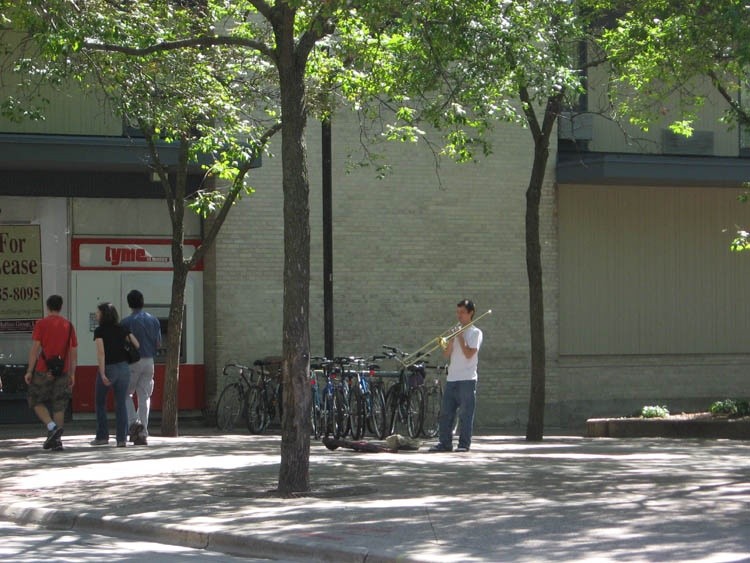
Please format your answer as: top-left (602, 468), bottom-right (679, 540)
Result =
top-left (400, 309), bottom-right (493, 368)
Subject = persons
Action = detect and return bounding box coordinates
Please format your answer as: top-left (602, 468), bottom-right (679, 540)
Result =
top-left (429, 300), bottom-right (483, 452)
top-left (25, 295), bottom-right (77, 451)
top-left (120, 290), bottom-right (162, 445)
top-left (90, 302), bottom-right (140, 446)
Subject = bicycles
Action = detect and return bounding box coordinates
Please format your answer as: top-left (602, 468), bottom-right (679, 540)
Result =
top-left (213, 344), bottom-right (459, 439)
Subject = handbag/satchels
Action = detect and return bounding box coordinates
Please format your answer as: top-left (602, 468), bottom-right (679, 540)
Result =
top-left (124, 328), bottom-right (140, 364)
top-left (46, 357), bottom-right (64, 378)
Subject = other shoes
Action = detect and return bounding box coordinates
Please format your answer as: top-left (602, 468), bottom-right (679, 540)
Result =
top-left (91, 439), bottom-right (108, 445)
top-left (428, 446), bottom-right (453, 452)
top-left (117, 442), bottom-right (126, 447)
top-left (455, 448), bottom-right (467, 452)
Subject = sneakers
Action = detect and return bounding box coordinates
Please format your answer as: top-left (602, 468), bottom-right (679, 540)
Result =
top-left (129, 422), bottom-right (144, 442)
top-left (134, 441), bottom-right (147, 445)
top-left (53, 441), bottom-right (62, 451)
top-left (43, 425), bottom-right (64, 449)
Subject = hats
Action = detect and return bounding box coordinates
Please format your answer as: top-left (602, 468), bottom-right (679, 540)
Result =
top-left (458, 299), bottom-right (474, 310)
